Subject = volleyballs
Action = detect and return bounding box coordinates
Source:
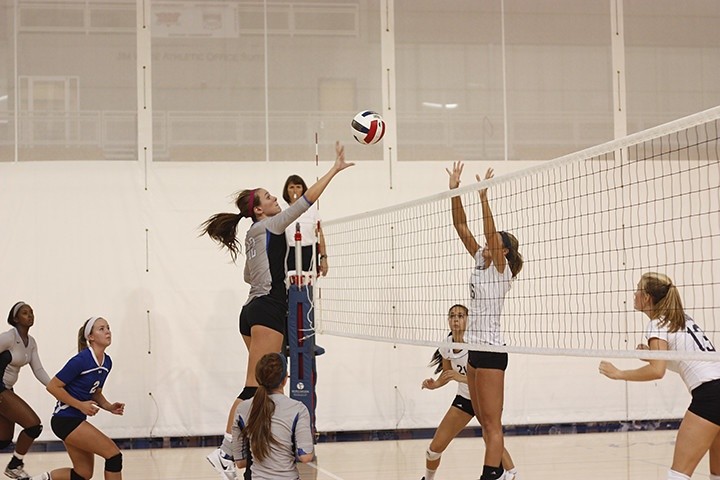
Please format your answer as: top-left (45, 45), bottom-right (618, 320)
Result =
top-left (350, 109), bottom-right (386, 145)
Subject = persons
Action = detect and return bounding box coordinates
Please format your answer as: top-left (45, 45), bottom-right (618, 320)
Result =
top-left (231, 353), bottom-right (315, 480)
top-left (17, 316), bottom-right (125, 480)
top-left (445, 160), bottom-right (524, 480)
top-left (599, 272), bottom-right (720, 480)
top-left (281, 174), bottom-right (328, 347)
top-left (421, 304), bottom-right (519, 480)
top-left (0, 301), bottom-right (51, 480)
top-left (206, 141), bottom-right (355, 480)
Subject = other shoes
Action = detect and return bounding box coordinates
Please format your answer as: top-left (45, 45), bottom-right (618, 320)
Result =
top-left (314, 344), bottom-right (325, 355)
top-left (32, 473), bottom-right (46, 480)
top-left (497, 469), bottom-right (515, 480)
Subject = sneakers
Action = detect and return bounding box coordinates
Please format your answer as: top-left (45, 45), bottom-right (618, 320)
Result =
top-left (206, 446), bottom-right (240, 480)
top-left (3, 465), bottom-right (32, 480)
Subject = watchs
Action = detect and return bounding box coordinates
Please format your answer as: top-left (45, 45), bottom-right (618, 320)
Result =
top-left (320, 255), bottom-right (327, 259)
